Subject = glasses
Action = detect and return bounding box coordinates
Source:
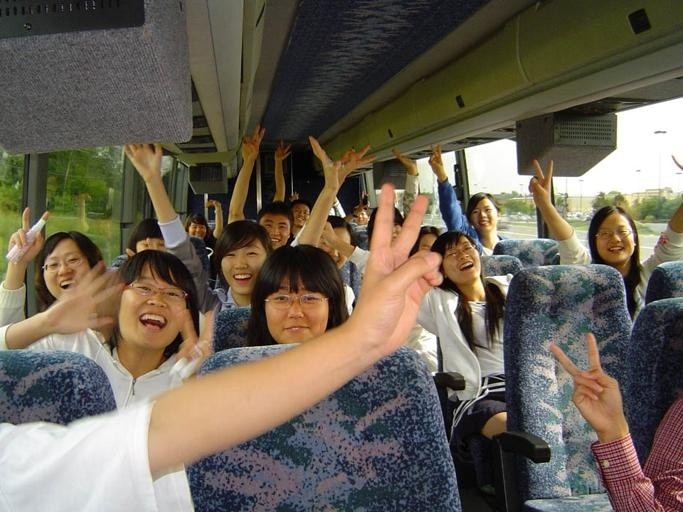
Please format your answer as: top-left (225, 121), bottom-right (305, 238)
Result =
top-left (445, 243), bottom-right (477, 260)
top-left (125, 281), bottom-right (188, 301)
top-left (264, 293), bottom-right (329, 309)
top-left (41, 255), bottom-right (83, 273)
top-left (595, 228), bottom-right (634, 238)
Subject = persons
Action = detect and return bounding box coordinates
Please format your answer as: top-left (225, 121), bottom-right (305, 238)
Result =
top-left (549, 332), bottom-right (683, 512)
top-left (269, 139), bottom-right (310, 243)
top-left (322, 222), bottom-right (515, 465)
top-left (428, 144), bottom-right (511, 256)
top-left (109, 217), bottom-right (163, 271)
top-left (321, 212), bottom-right (354, 314)
top-left (227, 125), bottom-right (294, 251)
top-left (245, 244), bottom-right (349, 345)
top-left (330, 192), bottom-right (370, 225)
top-left (0, 208), bottom-right (106, 328)
top-left (409, 226), bottom-right (440, 257)
top-left (187, 201), bottom-right (223, 247)
top-left (0, 183), bottom-right (444, 512)
top-left (364, 205), bottom-right (404, 251)
top-left (124, 135), bottom-right (341, 315)
top-left (528, 158), bottom-right (682, 328)
top-left (0, 249), bottom-right (212, 411)
top-left (289, 191), bottom-right (300, 201)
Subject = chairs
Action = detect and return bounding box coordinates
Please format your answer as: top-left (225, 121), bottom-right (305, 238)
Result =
top-left (190, 236), bottom-right (211, 275)
top-left (434, 255), bottom-right (522, 491)
top-left (489, 263), bottom-right (634, 512)
top-left (0, 350), bottom-right (118, 426)
top-left (110, 253), bottom-right (127, 270)
top-left (646, 260), bottom-right (683, 306)
top-left (340, 261), bottom-right (359, 298)
top-left (351, 223), bottom-right (369, 249)
top-left (494, 238), bottom-right (560, 267)
top-left (211, 308), bottom-right (256, 351)
top-left (626, 297), bottom-right (683, 469)
top-left (183, 342), bottom-right (462, 512)
